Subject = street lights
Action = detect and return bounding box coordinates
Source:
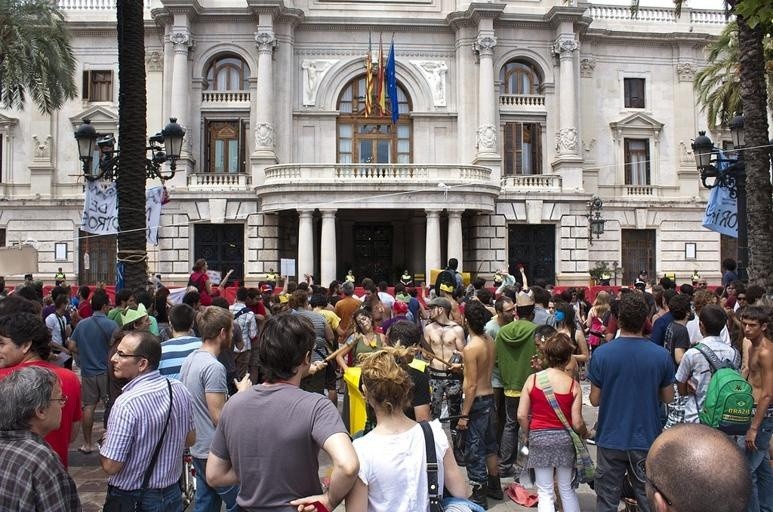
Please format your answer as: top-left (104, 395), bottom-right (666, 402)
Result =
top-left (75, 117), bottom-right (185, 211)
top-left (692, 112), bottom-right (748, 284)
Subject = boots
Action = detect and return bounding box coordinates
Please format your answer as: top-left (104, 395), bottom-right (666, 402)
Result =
top-left (486, 474), bottom-right (504, 499)
top-left (465, 484), bottom-right (488, 510)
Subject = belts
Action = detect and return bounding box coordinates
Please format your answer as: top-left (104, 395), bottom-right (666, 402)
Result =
top-left (430, 371), bottom-right (447, 377)
top-left (474, 394), bottom-right (496, 402)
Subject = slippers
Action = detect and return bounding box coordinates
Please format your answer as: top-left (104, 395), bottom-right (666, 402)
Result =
top-left (78, 444), bottom-right (91, 454)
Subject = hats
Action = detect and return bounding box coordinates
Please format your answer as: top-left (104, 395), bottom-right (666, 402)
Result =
top-left (440, 283), bottom-right (454, 292)
top-left (427, 298), bottom-right (452, 310)
top-left (260, 284), bottom-right (272, 292)
top-left (639, 270), bottom-right (647, 275)
top-left (120, 302), bottom-right (147, 326)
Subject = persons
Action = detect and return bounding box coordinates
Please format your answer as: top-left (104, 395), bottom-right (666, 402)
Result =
top-left (0, 267), bottom-right (91, 317)
top-left (178, 305), bottom-right (252, 512)
top-left (588, 289), bottom-right (610, 353)
top-left (664, 294), bottom-right (696, 364)
top-left (484, 296), bottom-right (515, 415)
top-left (608, 288), bottom-right (653, 340)
top-left (364, 30), bottom-right (374, 118)
top-left (309, 294), bottom-right (346, 408)
top-left (643, 423), bottom-right (751, 512)
top-left (336, 310), bottom-right (386, 433)
top-left (528, 285), bottom-right (554, 326)
top-left (345, 269), bottom-right (356, 284)
top-left (182, 292), bottom-right (200, 309)
top-left (67, 292), bottom-right (119, 453)
top-left (375, 34), bottom-right (386, 117)
top-left (102, 304), bottom-right (153, 436)
top-left (436, 258), bottom-right (463, 295)
top-left (335, 281), bottom-right (363, 394)
top-left (43, 295), bottom-right (73, 371)
top-left (675, 304), bottom-right (741, 439)
top-left (495, 293), bottom-right (539, 477)
top-left (0, 313), bottom-right (84, 472)
top-left (587, 296), bottom-right (675, 512)
top-left (344, 350), bottom-right (473, 512)
top-left (289, 290), bottom-right (334, 394)
top-left (458, 300), bottom-right (504, 511)
top-left (228, 287), bottom-right (257, 383)
top-left (516, 333), bottom-right (585, 512)
top-left (651, 289), bottom-right (678, 345)
top-left (154, 285), bottom-right (172, 331)
top-left (385, 36), bottom-right (399, 124)
top-left (423, 297), bottom-right (466, 466)
top-left (0, 367), bottom-right (82, 512)
top-left (361, 269), bottom-right (425, 322)
top-left (98, 330), bottom-right (197, 512)
top-left (260, 268), bottom-right (342, 311)
top-left (186, 259), bottom-right (224, 306)
top-left (247, 288), bottom-right (268, 375)
top-left (357, 320), bottom-right (431, 435)
top-left (635, 259), bottom-right (767, 319)
top-left (473, 265), bottom-right (588, 305)
top-left (530, 324), bottom-right (579, 384)
top-left (205, 314), bottom-right (360, 512)
top-left (155, 304), bottom-right (203, 381)
top-left (739, 306), bottom-right (773, 512)
top-left (552, 302), bottom-right (590, 362)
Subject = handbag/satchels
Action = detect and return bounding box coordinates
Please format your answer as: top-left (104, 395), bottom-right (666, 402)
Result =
top-left (103, 374), bottom-right (173, 511)
top-left (536, 371), bottom-right (596, 482)
top-left (418, 420), bottom-right (486, 512)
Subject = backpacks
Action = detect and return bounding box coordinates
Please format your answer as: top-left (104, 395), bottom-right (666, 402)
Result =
top-left (692, 342), bottom-right (754, 437)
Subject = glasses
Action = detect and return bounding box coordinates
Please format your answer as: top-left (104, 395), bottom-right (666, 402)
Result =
top-left (49, 394), bottom-right (68, 404)
top-left (638, 458), bottom-right (672, 505)
top-left (736, 296), bottom-right (746, 300)
top-left (114, 346), bottom-right (147, 359)
top-left (570, 293), bottom-right (576, 296)
